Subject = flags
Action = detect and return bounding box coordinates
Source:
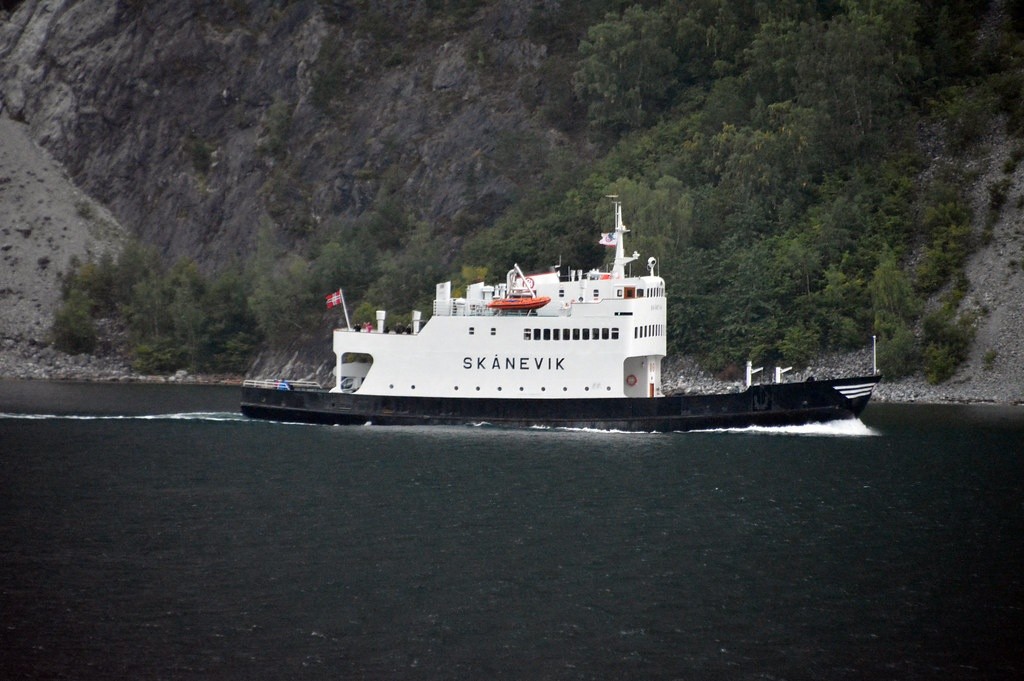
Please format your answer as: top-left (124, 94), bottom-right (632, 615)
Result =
top-left (325, 291), bottom-right (343, 310)
top-left (598, 232), bottom-right (618, 247)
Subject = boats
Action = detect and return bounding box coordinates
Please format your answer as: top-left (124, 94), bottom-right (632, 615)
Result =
top-left (237, 198), bottom-right (881, 429)
top-left (487, 297), bottom-right (550, 310)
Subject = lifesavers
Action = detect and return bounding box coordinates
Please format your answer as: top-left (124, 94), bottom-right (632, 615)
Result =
top-left (627, 375), bottom-right (637, 386)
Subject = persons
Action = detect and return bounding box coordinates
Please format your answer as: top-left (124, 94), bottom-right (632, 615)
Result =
top-left (362, 321), bottom-right (374, 333)
top-left (393, 322), bottom-right (413, 335)
top-left (353, 321), bottom-right (362, 332)
top-left (383, 325), bottom-right (391, 334)
